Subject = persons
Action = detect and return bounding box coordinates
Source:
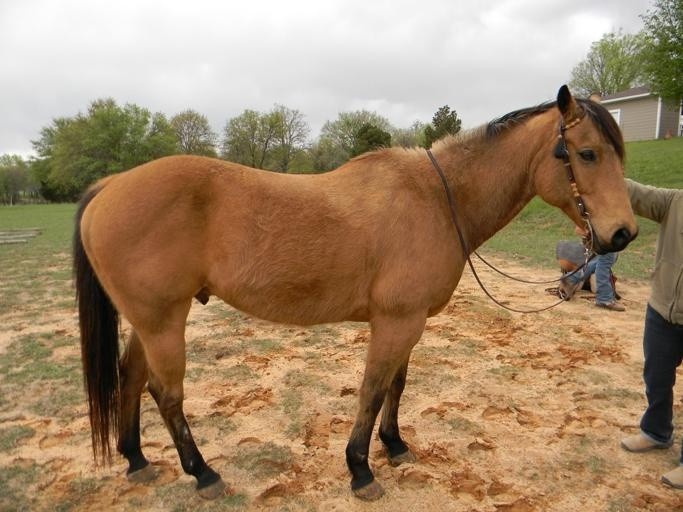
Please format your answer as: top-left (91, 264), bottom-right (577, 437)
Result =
top-left (620, 178), bottom-right (683, 489)
top-left (559, 252), bottom-right (626, 311)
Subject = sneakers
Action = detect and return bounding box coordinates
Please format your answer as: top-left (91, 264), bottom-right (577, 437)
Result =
top-left (622, 431), bottom-right (674, 452)
top-left (662, 465), bottom-right (683, 487)
top-left (597, 299), bottom-right (625, 311)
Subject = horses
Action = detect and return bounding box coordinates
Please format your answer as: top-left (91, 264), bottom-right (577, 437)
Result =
top-left (69, 83), bottom-right (639, 503)
